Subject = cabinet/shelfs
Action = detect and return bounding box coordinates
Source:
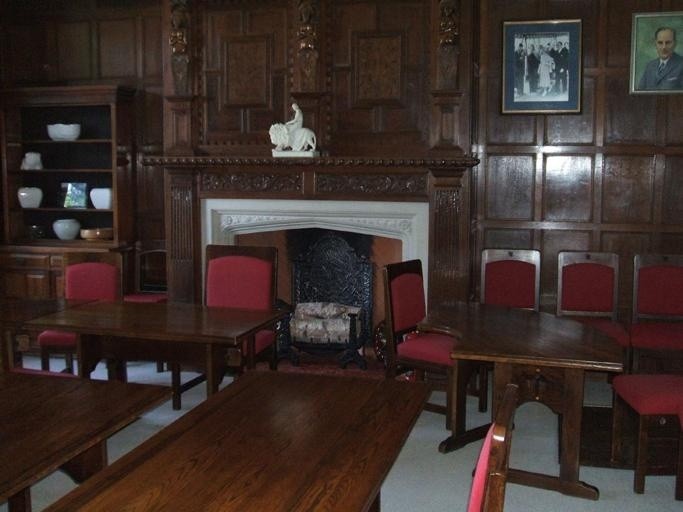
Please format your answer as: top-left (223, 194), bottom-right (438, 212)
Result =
top-left (0, 86), bottom-right (136, 357)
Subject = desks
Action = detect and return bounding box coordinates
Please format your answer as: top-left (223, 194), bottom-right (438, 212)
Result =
top-left (1, 295), bottom-right (435, 510)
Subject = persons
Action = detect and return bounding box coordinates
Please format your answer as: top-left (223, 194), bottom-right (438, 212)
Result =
top-left (283, 103), bottom-right (304, 149)
top-left (514, 41), bottom-right (569, 97)
top-left (638, 25), bottom-right (682, 91)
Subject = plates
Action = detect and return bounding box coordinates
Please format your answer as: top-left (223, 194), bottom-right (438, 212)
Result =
top-left (80, 228), bottom-right (114, 240)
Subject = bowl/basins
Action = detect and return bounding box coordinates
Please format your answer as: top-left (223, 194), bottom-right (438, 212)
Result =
top-left (48, 125), bottom-right (81, 140)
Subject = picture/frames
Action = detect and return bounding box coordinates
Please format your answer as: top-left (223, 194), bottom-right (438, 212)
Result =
top-left (630, 10), bottom-right (683, 96)
top-left (500, 17), bottom-right (583, 117)
